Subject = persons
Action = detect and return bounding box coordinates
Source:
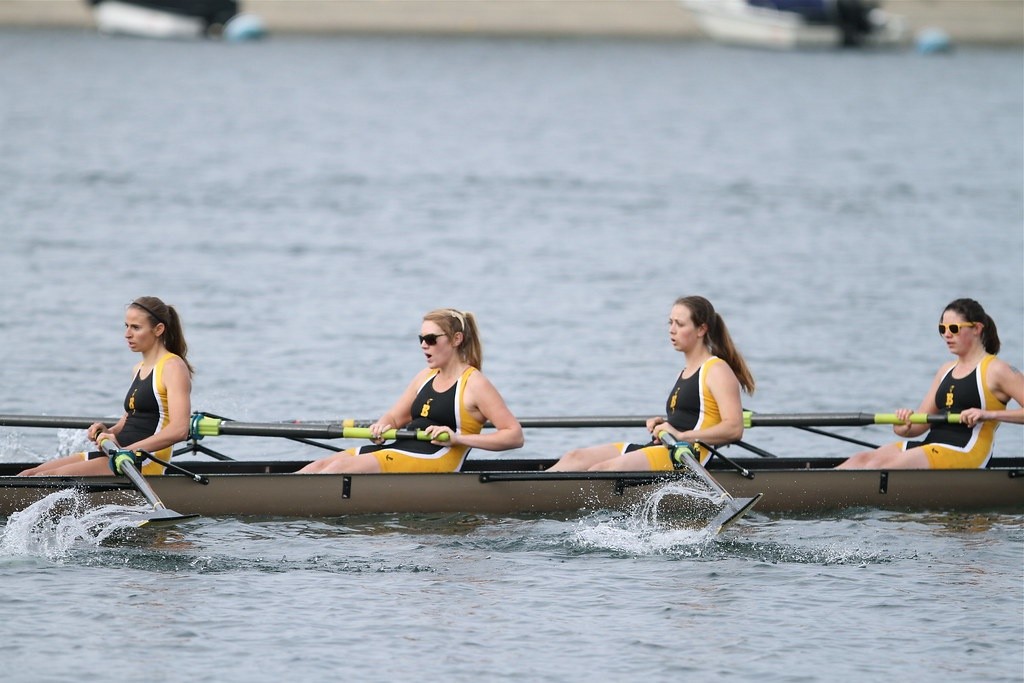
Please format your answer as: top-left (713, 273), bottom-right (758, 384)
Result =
top-left (833, 298), bottom-right (1024, 471)
top-left (545, 295), bottom-right (756, 473)
top-left (16, 296), bottom-right (195, 476)
top-left (295, 308), bottom-right (525, 474)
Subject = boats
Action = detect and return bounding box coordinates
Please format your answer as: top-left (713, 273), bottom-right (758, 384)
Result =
top-left (1, 456), bottom-right (1024, 520)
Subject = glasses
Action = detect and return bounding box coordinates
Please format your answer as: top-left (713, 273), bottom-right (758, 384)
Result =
top-left (938, 321), bottom-right (984, 335)
top-left (418, 333), bottom-right (454, 345)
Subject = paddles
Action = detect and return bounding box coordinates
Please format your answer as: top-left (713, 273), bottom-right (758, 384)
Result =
top-left (94, 429), bottom-right (200, 522)
top-left (657, 430), bottom-right (764, 536)
top-left (479, 409), bottom-right (964, 429)
top-left (0, 412), bottom-right (450, 443)
